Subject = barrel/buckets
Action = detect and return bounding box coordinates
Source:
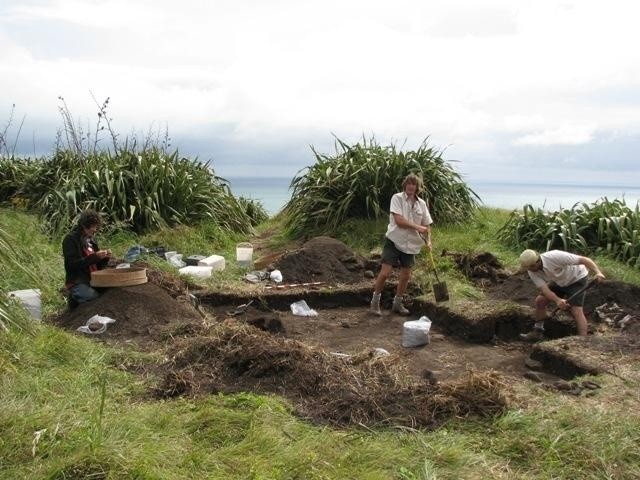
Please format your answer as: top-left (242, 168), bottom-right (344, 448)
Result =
top-left (236, 241), bottom-right (252, 261)
top-left (7, 288), bottom-right (43, 320)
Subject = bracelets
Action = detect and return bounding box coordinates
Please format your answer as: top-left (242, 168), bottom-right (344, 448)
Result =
top-left (415, 225), bottom-right (418, 230)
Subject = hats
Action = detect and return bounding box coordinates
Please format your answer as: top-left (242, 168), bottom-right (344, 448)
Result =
top-left (516, 249), bottom-right (539, 272)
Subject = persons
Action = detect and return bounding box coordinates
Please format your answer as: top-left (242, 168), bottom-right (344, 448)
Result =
top-left (60, 209), bottom-right (112, 303)
top-left (368, 175), bottom-right (434, 316)
top-left (518, 249), bottom-right (605, 342)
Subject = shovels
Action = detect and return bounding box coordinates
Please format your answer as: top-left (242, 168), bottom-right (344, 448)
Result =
top-left (528, 278), bottom-right (599, 322)
top-left (419, 232), bottom-right (449, 302)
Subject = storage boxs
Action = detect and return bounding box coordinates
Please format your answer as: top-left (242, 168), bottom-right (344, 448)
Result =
top-left (180, 266), bottom-right (212, 281)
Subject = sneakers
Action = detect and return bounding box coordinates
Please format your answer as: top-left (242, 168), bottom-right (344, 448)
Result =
top-left (518, 327), bottom-right (544, 342)
top-left (370, 301), bottom-right (381, 316)
top-left (392, 302), bottom-right (410, 316)
